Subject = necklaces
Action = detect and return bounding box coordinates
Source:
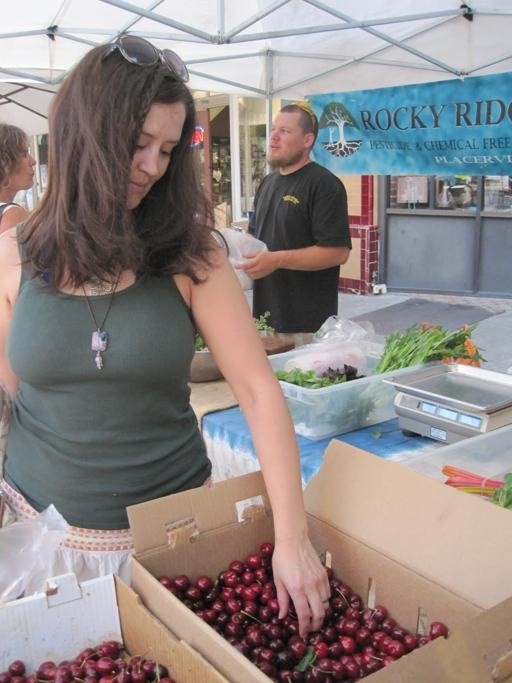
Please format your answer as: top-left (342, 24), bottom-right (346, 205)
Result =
top-left (77, 268), bottom-right (122, 372)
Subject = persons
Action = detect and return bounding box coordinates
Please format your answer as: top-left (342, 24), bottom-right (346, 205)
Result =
top-left (0, 36), bottom-right (332, 639)
top-left (235, 105), bottom-right (352, 345)
top-left (0, 122), bottom-right (37, 236)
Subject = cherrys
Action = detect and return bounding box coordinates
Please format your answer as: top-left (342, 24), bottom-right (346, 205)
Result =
top-left (157, 540), bottom-right (448, 683)
top-left (0, 642), bottom-right (174, 682)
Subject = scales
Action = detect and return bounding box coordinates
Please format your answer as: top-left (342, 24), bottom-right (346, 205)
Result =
top-left (381, 361), bottom-right (512, 445)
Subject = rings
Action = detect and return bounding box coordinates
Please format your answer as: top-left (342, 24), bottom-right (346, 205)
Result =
top-left (322, 599), bottom-right (329, 603)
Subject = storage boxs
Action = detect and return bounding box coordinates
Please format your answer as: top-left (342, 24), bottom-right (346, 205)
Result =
top-left (266, 341), bottom-right (445, 440)
top-left (0, 437), bottom-right (511, 682)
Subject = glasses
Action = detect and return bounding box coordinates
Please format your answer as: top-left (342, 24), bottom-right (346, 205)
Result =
top-left (293, 101), bottom-right (316, 132)
top-left (103, 34), bottom-right (189, 83)
